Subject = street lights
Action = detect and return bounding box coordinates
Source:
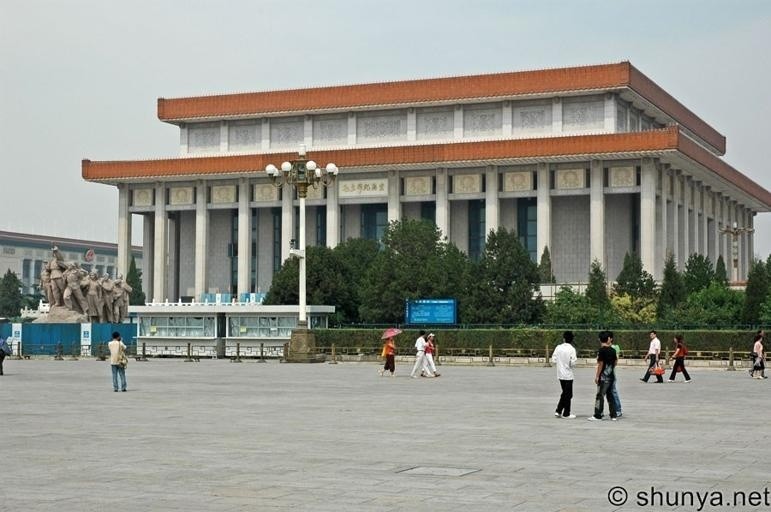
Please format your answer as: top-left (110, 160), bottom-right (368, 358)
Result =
top-left (718, 221), bottom-right (755, 281)
top-left (265, 145), bottom-right (339, 365)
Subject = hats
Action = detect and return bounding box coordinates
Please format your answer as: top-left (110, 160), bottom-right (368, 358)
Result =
top-left (427, 332), bottom-right (435, 339)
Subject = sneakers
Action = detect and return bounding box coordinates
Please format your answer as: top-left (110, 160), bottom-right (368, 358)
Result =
top-left (639, 377), bottom-right (647, 382)
top-left (685, 379), bottom-right (693, 383)
top-left (555, 410), bottom-right (563, 417)
top-left (749, 369), bottom-right (754, 377)
top-left (434, 373), bottom-right (441, 377)
top-left (588, 415), bottom-right (603, 421)
top-left (609, 411), bottom-right (623, 417)
top-left (655, 380), bottom-right (663, 383)
top-left (761, 374), bottom-right (768, 378)
top-left (667, 379), bottom-right (675, 382)
top-left (611, 416), bottom-right (618, 421)
top-left (561, 413), bottom-right (577, 419)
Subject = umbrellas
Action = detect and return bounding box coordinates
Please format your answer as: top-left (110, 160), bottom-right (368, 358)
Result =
top-left (381, 329), bottom-right (403, 340)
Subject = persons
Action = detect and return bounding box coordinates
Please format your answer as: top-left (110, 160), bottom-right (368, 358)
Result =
top-left (640, 331), bottom-right (663, 384)
top-left (588, 331), bottom-right (617, 421)
top-left (753, 337), bottom-right (764, 379)
top-left (378, 337), bottom-right (395, 378)
top-left (601, 331), bottom-right (622, 417)
top-left (552, 331), bottom-right (577, 419)
top-left (668, 334), bottom-right (692, 383)
top-left (421, 333), bottom-right (441, 377)
top-left (0, 335), bottom-right (11, 375)
top-left (108, 332), bottom-right (127, 393)
top-left (409, 330), bottom-right (435, 378)
top-left (751, 330), bottom-right (768, 379)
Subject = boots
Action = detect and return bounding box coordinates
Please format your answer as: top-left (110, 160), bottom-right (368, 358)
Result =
top-left (753, 370), bottom-right (757, 378)
top-left (757, 371), bottom-right (763, 379)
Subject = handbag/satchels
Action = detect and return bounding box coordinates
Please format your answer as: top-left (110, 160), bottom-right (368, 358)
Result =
top-left (750, 352), bottom-right (758, 357)
top-left (381, 346), bottom-right (387, 358)
top-left (117, 340), bottom-right (128, 370)
top-left (676, 365), bottom-right (682, 372)
top-left (655, 361), bottom-right (665, 375)
